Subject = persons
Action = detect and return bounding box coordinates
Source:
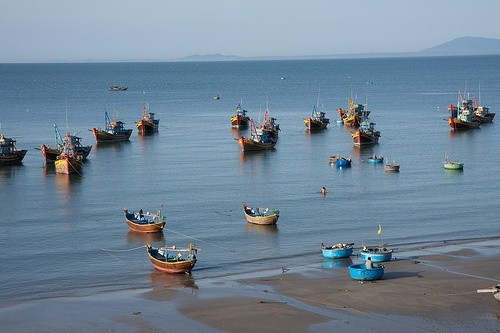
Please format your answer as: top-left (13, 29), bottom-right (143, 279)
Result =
top-left (365, 256), bottom-right (373, 269)
top-left (321, 187), bottom-right (326, 194)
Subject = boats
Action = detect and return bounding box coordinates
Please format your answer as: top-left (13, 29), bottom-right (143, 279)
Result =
top-left (477, 285), bottom-right (500, 301)
top-left (442, 155), bottom-right (464, 169)
top-left (335, 89), bottom-right (380, 147)
top-left (447, 80), bottom-right (497, 131)
top-left (234, 102), bottom-right (281, 152)
top-left (243, 205), bottom-right (281, 225)
top-left (383, 163), bottom-right (401, 171)
top-left (145, 243), bottom-right (200, 275)
top-left (122, 205), bottom-right (168, 233)
top-left (303, 88), bottom-right (330, 133)
top-left (88, 107), bottom-right (134, 142)
top-left (366, 153), bottom-right (384, 164)
top-left (230, 98), bottom-right (250, 130)
top-left (214, 96), bottom-right (219, 99)
top-left (280, 77), bottom-right (285, 80)
top-left (347, 263), bottom-right (386, 281)
top-left (134, 91), bottom-right (161, 134)
top-left (328, 155), bottom-right (353, 167)
top-left (320, 243), bottom-right (355, 259)
top-left (0, 133), bottom-right (27, 164)
top-left (33, 102), bottom-right (94, 175)
top-left (107, 86), bottom-right (128, 91)
top-left (358, 245), bottom-right (393, 262)
